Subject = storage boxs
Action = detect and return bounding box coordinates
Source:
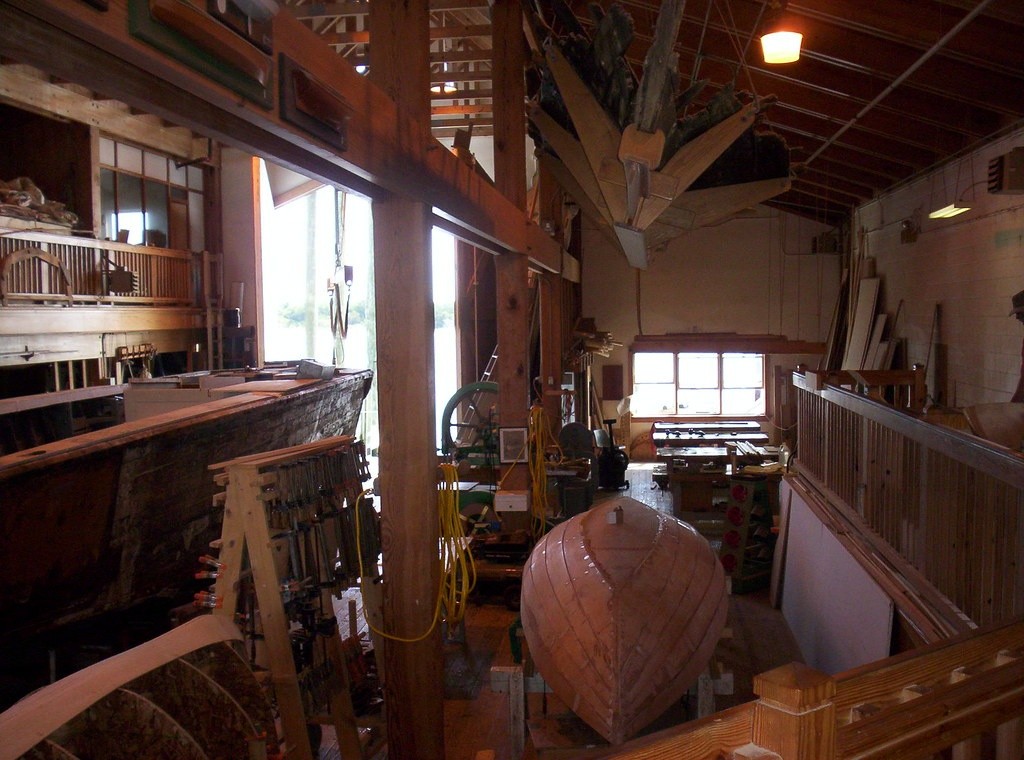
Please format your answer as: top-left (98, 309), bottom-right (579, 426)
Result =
top-left (566, 472), bottom-right (594, 512)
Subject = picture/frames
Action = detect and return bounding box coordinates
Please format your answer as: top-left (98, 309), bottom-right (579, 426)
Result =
top-left (498, 426), bottom-right (529, 464)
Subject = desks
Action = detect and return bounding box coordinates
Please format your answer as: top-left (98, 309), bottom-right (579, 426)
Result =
top-left (652, 420), bottom-right (780, 521)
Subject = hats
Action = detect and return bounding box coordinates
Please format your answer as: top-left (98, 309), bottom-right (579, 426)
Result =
top-left (1009, 289), bottom-right (1024, 317)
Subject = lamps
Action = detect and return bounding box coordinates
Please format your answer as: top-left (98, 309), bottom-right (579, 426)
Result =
top-left (760, 31), bottom-right (803, 64)
top-left (928, 200), bottom-right (975, 219)
